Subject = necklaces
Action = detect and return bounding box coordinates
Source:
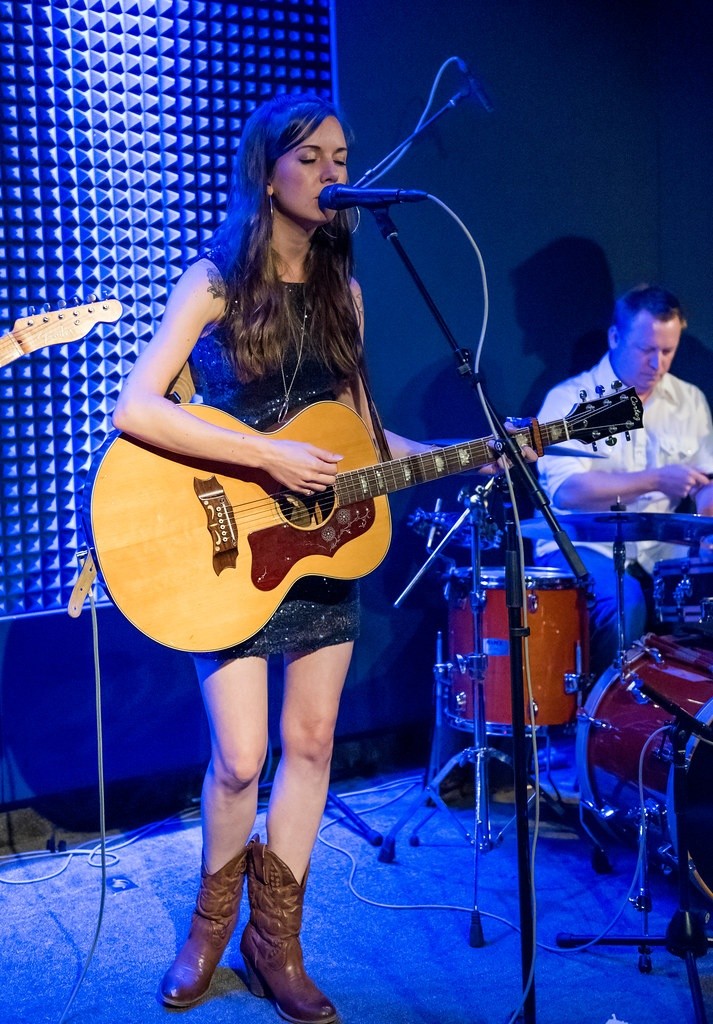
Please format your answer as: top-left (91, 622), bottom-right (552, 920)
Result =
top-left (263, 265), bottom-right (314, 427)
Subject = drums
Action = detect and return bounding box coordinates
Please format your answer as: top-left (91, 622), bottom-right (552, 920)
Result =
top-left (653, 557), bottom-right (713, 625)
top-left (445, 564), bottom-right (592, 737)
top-left (575, 633), bottom-right (713, 900)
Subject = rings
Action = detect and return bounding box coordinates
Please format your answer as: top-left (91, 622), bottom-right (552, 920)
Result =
top-left (304, 489), bottom-right (311, 497)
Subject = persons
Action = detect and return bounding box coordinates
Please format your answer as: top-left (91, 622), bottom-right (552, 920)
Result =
top-left (537, 286), bottom-right (713, 669)
top-left (112, 97), bottom-right (543, 1024)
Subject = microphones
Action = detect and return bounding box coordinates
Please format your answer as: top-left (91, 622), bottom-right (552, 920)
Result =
top-left (456, 57), bottom-right (494, 113)
top-left (318, 183), bottom-right (429, 210)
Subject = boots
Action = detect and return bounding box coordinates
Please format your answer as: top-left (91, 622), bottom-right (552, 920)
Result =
top-left (158, 843), bottom-right (248, 1005)
top-left (238, 844), bottom-right (339, 1024)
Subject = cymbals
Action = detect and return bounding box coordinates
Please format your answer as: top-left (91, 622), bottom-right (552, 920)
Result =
top-left (418, 439), bottom-right (606, 459)
top-left (506, 511), bottom-right (713, 543)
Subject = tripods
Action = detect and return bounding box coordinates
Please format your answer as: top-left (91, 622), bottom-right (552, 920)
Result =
top-left (374, 466), bottom-right (578, 865)
top-left (554, 677), bottom-right (713, 1024)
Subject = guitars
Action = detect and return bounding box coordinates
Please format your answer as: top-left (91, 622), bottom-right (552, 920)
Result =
top-left (87, 379), bottom-right (644, 654)
top-left (0, 290), bottom-right (123, 368)
